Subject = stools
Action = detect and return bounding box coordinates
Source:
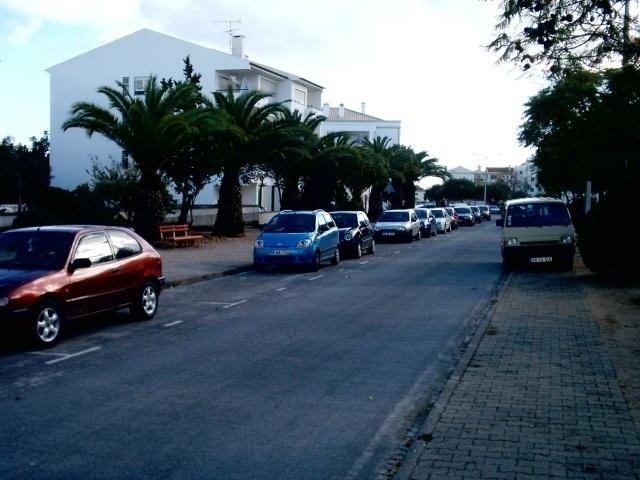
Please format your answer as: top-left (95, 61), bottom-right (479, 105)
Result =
top-left (193, 231), bottom-right (213, 240)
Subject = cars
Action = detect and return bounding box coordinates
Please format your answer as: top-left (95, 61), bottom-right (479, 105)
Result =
top-left (1, 223), bottom-right (166, 349)
top-left (375, 204), bottom-right (500, 244)
top-left (253, 208), bottom-right (341, 274)
top-left (328, 210), bottom-right (376, 260)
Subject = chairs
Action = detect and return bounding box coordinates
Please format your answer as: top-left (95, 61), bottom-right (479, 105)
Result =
top-left (159, 224), bottom-right (204, 249)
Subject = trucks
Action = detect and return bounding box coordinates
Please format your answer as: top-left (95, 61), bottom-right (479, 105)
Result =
top-left (495, 196), bottom-right (578, 273)
